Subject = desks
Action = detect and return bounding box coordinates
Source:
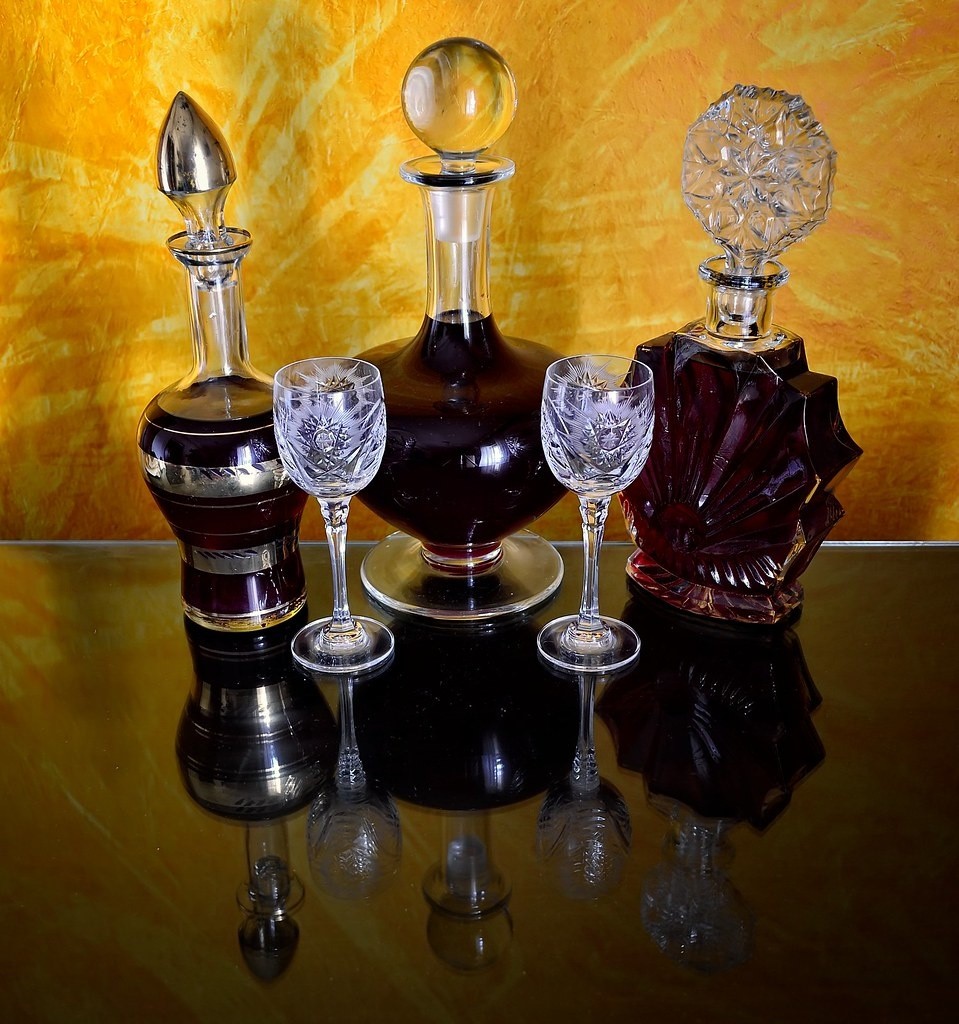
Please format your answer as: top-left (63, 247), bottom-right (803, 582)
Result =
top-left (0, 539), bottom-right (959, 1024)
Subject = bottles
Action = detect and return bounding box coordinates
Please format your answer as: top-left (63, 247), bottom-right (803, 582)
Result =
top-left (175, 618), bottom-right (338, 984)
top-left (596, 579), bottom-right (824, 971)
top-left (614, 84), bottom-right (863, 625)
top-left (348, 36), bottom-right (567, 621)
top-left (336, 564), bottom-right (583, 969)
top-left (135, 91), bottom-right (309, 633)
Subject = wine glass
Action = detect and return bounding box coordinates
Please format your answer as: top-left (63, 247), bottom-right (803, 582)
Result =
top-left (537, 647), bottom-right (638, 897)
top-left (273, 359), bottom-right (394, 672)
top-left (537, 355), bottom-right (655, 672)
top-left (293, 651), bottom-right (400, 902)
